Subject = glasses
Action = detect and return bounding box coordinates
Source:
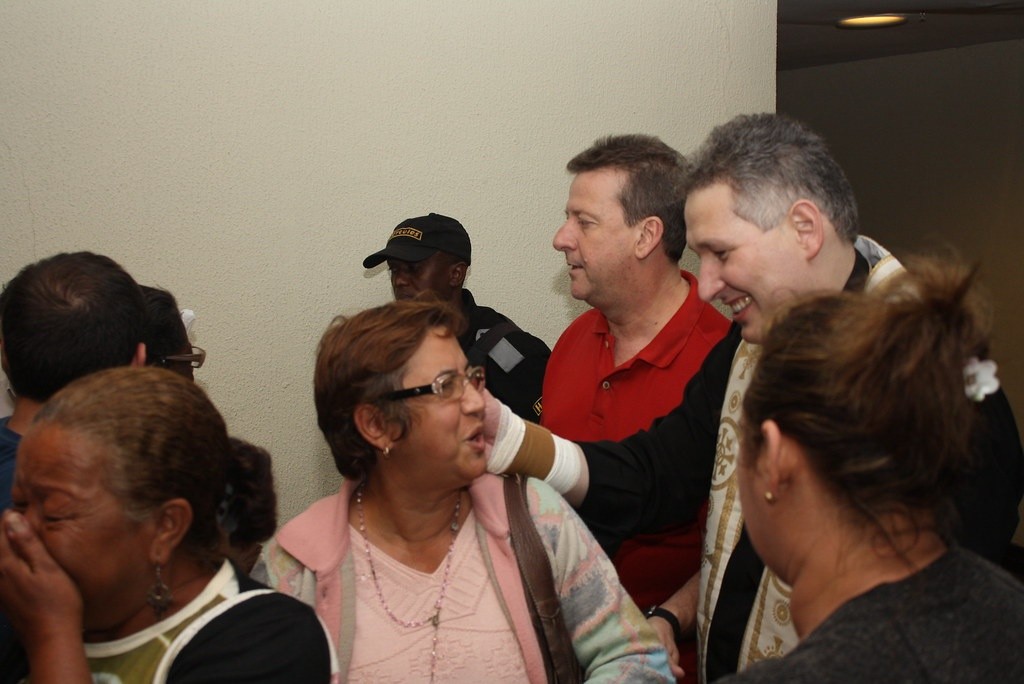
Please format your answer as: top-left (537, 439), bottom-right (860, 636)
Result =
top-left (163, 347), bottom-right (205, 367)
top-left (388, 366), bottom-right (485, 402)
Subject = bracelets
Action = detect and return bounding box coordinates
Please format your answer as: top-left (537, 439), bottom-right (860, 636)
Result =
top-left (645, 606), bottom-right (681, 641)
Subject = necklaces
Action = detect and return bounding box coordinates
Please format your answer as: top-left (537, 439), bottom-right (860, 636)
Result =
top-left (356, 488), bottom-right (462, 627)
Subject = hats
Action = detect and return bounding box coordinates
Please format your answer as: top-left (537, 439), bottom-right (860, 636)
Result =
top-left (363, 214), bottom-right (470, 269)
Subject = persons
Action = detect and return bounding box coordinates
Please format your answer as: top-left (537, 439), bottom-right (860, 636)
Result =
top-left (481, 115), bottom-right (914, 684)
top-left (713, 254), bottom-right (1023, 684)
top-left (542, 133), bottom-right (732, 684)
top-left (0, 251), bottom-right (339, 684)
top-left (363, 214), bottom-right (553, 424)
top-left (251, 305), bottom-right (672, 684)
